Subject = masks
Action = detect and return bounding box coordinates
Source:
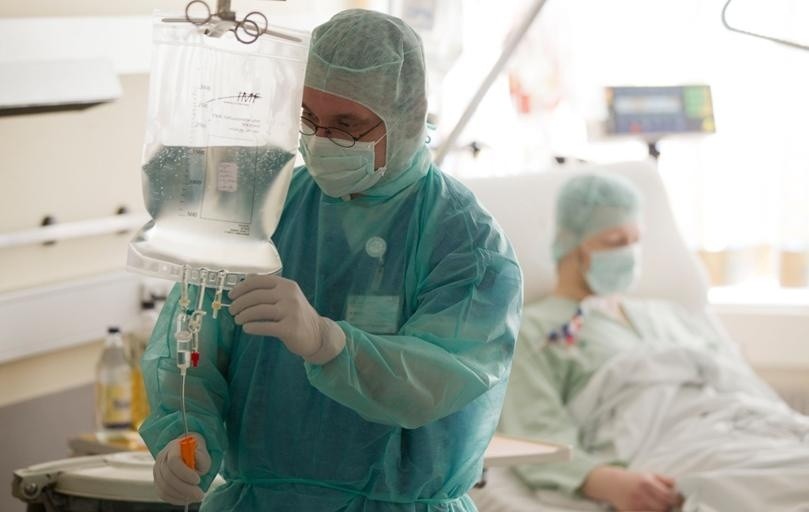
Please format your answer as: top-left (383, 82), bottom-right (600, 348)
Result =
top-left (299, 134), bottom-right (386, 198)
top-left (578, 243), bottom-right (642, 294)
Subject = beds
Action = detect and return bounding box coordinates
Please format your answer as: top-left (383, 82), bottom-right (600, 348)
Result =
top-left (456, 159), bottom-right (718, 512)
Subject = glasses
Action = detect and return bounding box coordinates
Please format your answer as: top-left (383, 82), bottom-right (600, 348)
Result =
top-left (299, 116), bottom-right (384, 148)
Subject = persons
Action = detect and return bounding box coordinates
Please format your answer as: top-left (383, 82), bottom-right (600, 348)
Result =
top-left (140, 6), bottom-right (527, 512)
top-left (506, 173), bottom-right (809, 512)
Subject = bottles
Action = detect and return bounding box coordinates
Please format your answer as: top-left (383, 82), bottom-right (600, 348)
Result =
top-left (95, 326), bottom-right (133, 439)
top-left (133, 302), bottom-right (156, 433)
